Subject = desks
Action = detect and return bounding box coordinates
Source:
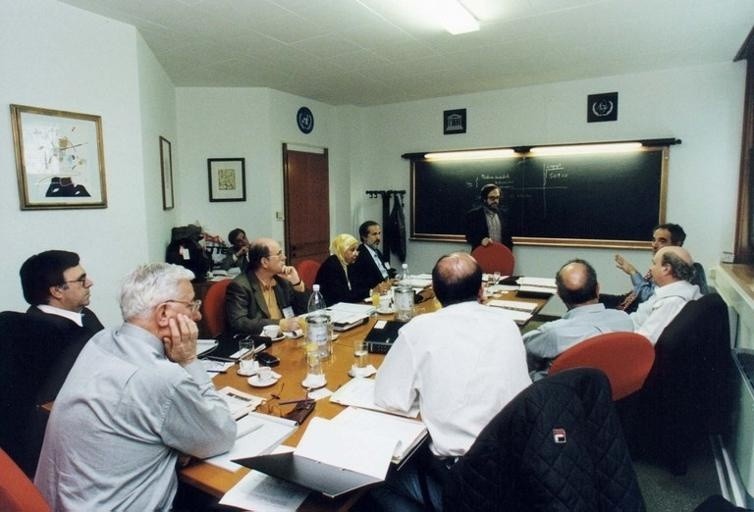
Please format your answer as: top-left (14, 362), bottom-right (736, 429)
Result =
top-left (156, 268), bottom-right (576, 511)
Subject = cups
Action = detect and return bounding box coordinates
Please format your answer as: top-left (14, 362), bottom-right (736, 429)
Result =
top-left (237, 272), bottom-right (503, 389)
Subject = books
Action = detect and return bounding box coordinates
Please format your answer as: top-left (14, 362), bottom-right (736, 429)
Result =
top-left (230, 406), bottom-right (429, 500)
top-left (361, 319), bottom-right (406, 353)
top-left (206, 332), bottom-right (272, 364)
top-left (332, 317), bottom-right (369, 332)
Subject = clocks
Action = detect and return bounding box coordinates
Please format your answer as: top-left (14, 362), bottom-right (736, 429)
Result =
top-left (296, 107), bottom-right (317, 137)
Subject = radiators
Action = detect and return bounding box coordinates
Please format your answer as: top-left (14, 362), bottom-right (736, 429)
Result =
top-left (729, 346), bottom-right (754, 512)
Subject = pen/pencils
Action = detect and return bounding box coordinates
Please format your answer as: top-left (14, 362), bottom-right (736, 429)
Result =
top-left (207, 370), bottom-right (227, 374)
top-left (235, 424), bottom-right (264, 440)
top-left (279, 399), bottom-right (315, 405)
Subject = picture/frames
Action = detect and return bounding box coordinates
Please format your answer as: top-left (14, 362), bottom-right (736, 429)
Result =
top-left (207, 158), bottom-right (248, 202)
top-left (10, 104), bottom-right (109, 210)
top-left (160, 136), bottom-right (176, 209)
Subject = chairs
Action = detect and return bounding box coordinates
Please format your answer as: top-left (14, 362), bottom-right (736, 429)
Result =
top-left (1, 447), bottom-right (57, 511)
top-left (548, 330), bottom-right (657, 429)
top-left (294, 259), bottom-right (326, 305)
top-left (652, 295), bottom-right (738, 452)
top-left (471, 242), bottom-right (516, 277)
top-left (204, 279), bottom-right (245, 342)
top-left (443, 367), bottom-right (632, 511)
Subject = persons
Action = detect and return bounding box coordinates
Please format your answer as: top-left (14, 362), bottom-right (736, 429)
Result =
top-left (312, 233), bottom-right (391, 307)
top-left (599, 223), bottom-right (686, 314)
top-left (629, 244), bottom-right (703, 346)
top-left (464, 183), bottom-right (514, 253)
top-left (218, 228), bottom-right (250, 269)
top-left (357, 251), bottom-right (533, 512)
top-left (224, 237), bottom-right (316, 338)
top-left (351, 220), bottom-right (399, 288)
top-left (33, 262), bottom-right (238, 512)
top-left (8, 250), bottom-right (106, 403)
top-left (521, 258), bottom-right (636, 382)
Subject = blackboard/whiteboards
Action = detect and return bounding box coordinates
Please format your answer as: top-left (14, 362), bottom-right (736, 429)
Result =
top-left (409, 138), bottom-right (670, 252)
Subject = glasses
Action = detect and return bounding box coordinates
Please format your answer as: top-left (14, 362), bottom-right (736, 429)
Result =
top-left (165, 297), bottom-right (204, 314)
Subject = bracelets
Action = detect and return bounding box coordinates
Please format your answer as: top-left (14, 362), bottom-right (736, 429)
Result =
top-left (291, 278), bottom-right (301, 286)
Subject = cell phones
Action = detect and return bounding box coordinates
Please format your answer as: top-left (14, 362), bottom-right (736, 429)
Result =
top-left (257, 352), bottom-right (280, 366)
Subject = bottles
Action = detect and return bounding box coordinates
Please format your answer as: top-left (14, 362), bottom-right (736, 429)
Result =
top-left (398, 264), bottom-right (412, 291)
